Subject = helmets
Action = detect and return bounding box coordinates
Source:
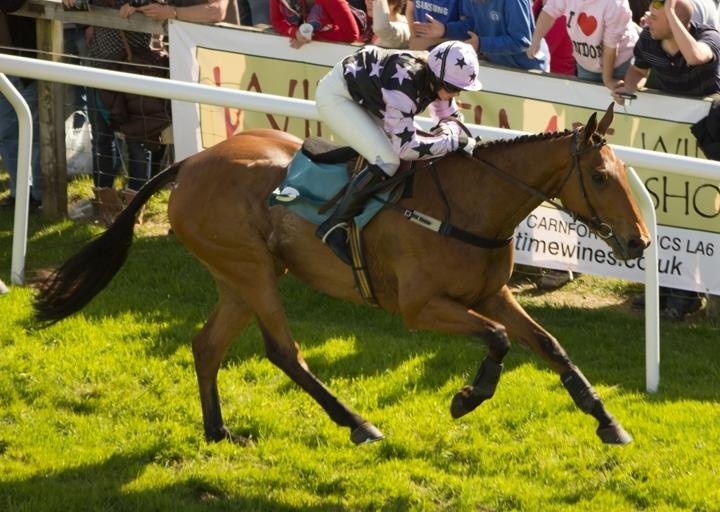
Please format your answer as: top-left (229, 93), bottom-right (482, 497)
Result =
top-left (426, 40), bottom-right (482, 92)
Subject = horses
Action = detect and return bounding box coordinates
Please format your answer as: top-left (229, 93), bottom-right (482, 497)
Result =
top-left (26, 101), bottom-right (651, 447)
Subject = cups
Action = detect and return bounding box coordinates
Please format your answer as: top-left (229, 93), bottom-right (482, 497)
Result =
top-left (298, 22), bottom-right (315, 42)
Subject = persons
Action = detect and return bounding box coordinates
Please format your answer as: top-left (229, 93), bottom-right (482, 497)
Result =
top-left (614, 0), bottom-right (719, 320)
top-left (413, 1), bottom-right (551, 72)
top-left (405, 1), bottom-right (459, 50)
top-left (364, 0), bottom-right (411, 49)
top-left (314, 40), bottom-right (482, 266)
top-left (2, 0), bottom-right (377, 218)
top-left (527, 0), bottom-right (639, 90)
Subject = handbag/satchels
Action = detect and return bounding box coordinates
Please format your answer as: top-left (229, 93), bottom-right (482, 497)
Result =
top-left (348, 6), bottom-right (374, 42)
top-left (123, 69), bottom-right (165, 115)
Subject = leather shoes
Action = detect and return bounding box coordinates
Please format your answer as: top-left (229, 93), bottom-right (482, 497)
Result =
top-left (666, 293), bottom-right (700, 320)
top-left (630, 288), bottom-right (670, 308)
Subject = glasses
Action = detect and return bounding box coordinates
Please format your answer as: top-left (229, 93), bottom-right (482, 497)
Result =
top-left (443, 82), bottom-right (461, 93)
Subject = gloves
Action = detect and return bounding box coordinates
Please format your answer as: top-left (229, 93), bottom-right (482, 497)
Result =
top-left (429, 123), bottom-right (453, 137)
top-left (457, 135), bottom-right (476, 158)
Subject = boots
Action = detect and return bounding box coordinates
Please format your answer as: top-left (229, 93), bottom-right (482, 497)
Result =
top-left (315, 164), bottom-right (391, 266)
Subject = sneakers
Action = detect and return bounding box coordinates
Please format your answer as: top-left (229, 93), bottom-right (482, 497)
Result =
top-left (538, 271), bottom-right (573, 288)
